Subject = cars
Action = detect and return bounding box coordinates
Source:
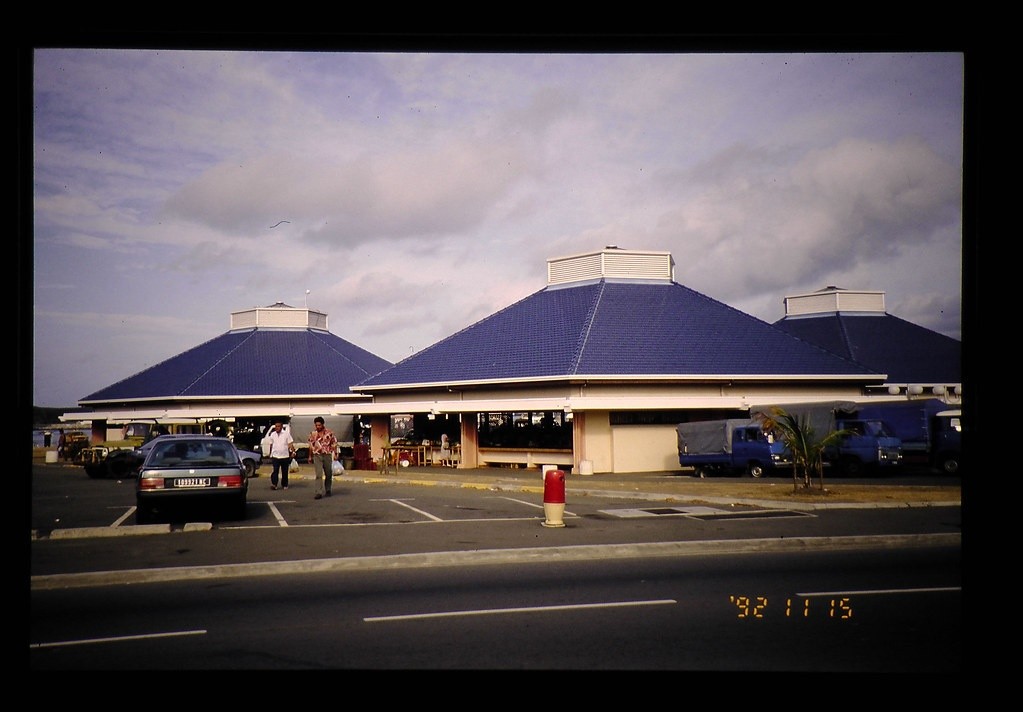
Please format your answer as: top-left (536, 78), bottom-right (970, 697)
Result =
top-left (134, 432), bottom-right (262, 481)
top-left (134, 437), bottom-right (250, 521)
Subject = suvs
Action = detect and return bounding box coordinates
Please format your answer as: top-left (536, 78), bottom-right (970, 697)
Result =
top-left (79, 416), bottom-right (240, 481)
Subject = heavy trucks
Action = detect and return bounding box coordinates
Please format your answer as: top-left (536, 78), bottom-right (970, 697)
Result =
top-left (841, 398), bottom-right (961, 475)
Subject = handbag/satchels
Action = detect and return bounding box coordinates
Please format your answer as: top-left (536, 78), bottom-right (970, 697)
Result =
top-left (332, 460), bottom-right (344, 476)
top-left (290, 458), bottom-right (300, 473)
top-left (443, 442), bottom-right (449, 449)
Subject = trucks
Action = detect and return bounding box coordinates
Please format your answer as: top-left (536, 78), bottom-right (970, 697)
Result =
top-left (750, 402), bottom-right (903, 477)
top-left (675, 420), bottom-right (793, 481)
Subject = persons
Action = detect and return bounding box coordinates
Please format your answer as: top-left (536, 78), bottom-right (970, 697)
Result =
top-left (57, 428), bottom-right (67, 461)
top-left (43, 430), bottom-right (52, 447)
top-left (308, 417), bottom-right (337, 504)
top-left (269, 421), bottom-right (294, 490)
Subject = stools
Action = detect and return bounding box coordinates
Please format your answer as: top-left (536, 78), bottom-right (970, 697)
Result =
top-left (418, 446), bottom-right (433, 465)
top-left (452, 446), bottom-right (461, 468)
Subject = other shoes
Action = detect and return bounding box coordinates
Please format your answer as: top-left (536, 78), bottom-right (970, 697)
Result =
top-left (324, 493), bottom-right (332, 497)
top-left (270, 484), bottom-right (277, 490)
top-left (281, 486), bottom-right (288, 490)
top-left (314, 494), bottom-right (322, 499)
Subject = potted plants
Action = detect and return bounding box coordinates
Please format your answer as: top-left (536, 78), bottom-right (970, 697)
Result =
top-left (342, 447), bottom-right (354, 470)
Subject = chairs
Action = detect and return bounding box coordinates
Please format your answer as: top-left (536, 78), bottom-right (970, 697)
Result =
top-left (210, 449), bottom-right (226, 459)
